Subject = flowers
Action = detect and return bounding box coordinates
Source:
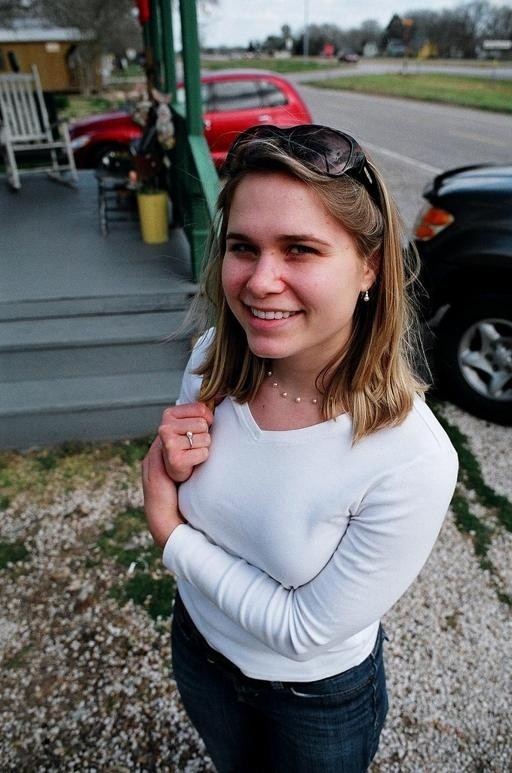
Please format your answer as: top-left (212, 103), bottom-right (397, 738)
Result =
top-left (125, 151), bottom-right (164, 193)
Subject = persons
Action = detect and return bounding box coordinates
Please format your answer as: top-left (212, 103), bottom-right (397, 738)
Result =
top-left (141, 121), bottom-right (461, 772)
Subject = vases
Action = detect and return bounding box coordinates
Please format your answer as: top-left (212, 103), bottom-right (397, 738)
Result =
top-left (134, 188), bottom-right (173, 243)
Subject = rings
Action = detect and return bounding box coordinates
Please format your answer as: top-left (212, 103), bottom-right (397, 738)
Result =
top-left (186, 431), bottom-right (194, 450)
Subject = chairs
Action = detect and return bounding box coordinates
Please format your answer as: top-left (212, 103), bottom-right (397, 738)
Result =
top-left (1, 61), bottom-right (80, 191)
top-left (94, 105), bottom-right (165, 237)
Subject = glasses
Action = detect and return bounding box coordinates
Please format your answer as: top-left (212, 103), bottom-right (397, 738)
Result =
top-left (225, 124), bottom-right (380, 203)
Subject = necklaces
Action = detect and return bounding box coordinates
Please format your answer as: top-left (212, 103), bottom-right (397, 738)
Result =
top-left (264, 367), bottom-right (336, 404)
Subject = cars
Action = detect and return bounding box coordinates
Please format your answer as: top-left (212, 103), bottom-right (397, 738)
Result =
top-left (66, 68), bottom-right (313, 179)
top-left (404, 163), bottom-right (511, 429)
top-left (338, 39), bottom-right (404, 62)
